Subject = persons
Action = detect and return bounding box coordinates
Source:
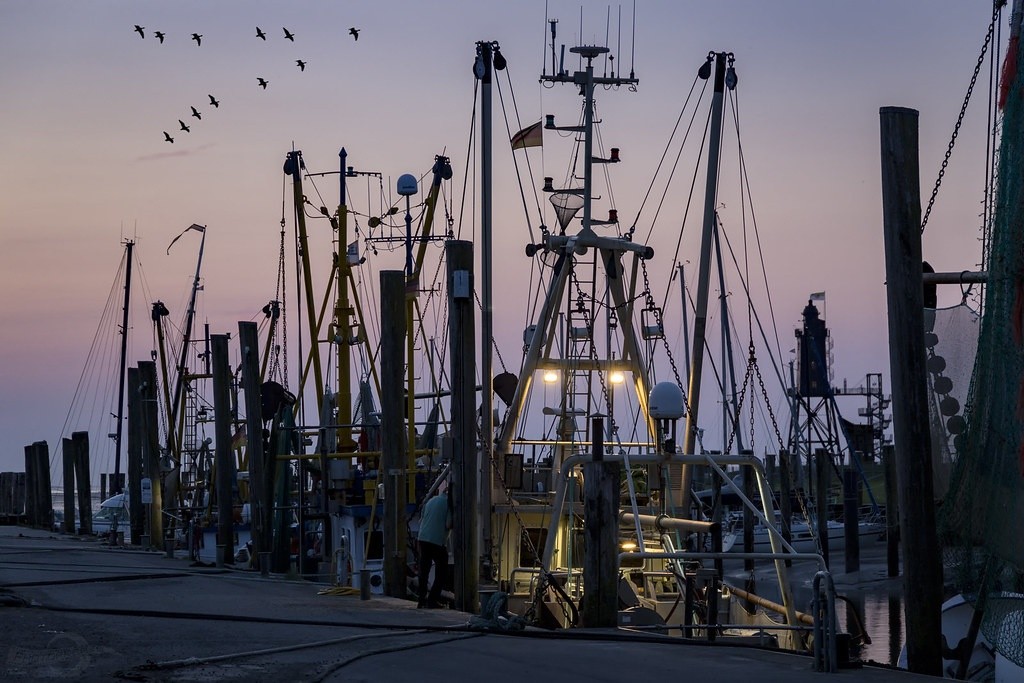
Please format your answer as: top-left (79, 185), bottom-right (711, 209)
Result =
top-left (416, 489), bottom-right (453, 610)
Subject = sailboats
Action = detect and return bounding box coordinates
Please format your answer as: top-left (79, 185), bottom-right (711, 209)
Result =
top-left (48, 0), bottom-right (885, 650)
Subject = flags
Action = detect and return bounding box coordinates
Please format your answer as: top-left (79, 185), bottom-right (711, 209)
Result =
top-left (512, 121), bottom-right (544, 150)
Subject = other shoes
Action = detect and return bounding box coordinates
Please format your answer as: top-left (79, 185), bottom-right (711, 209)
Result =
top-left (417, 596), bottom-right (428, 609)
top-left (427, 598), bottom-right (445, 610)
